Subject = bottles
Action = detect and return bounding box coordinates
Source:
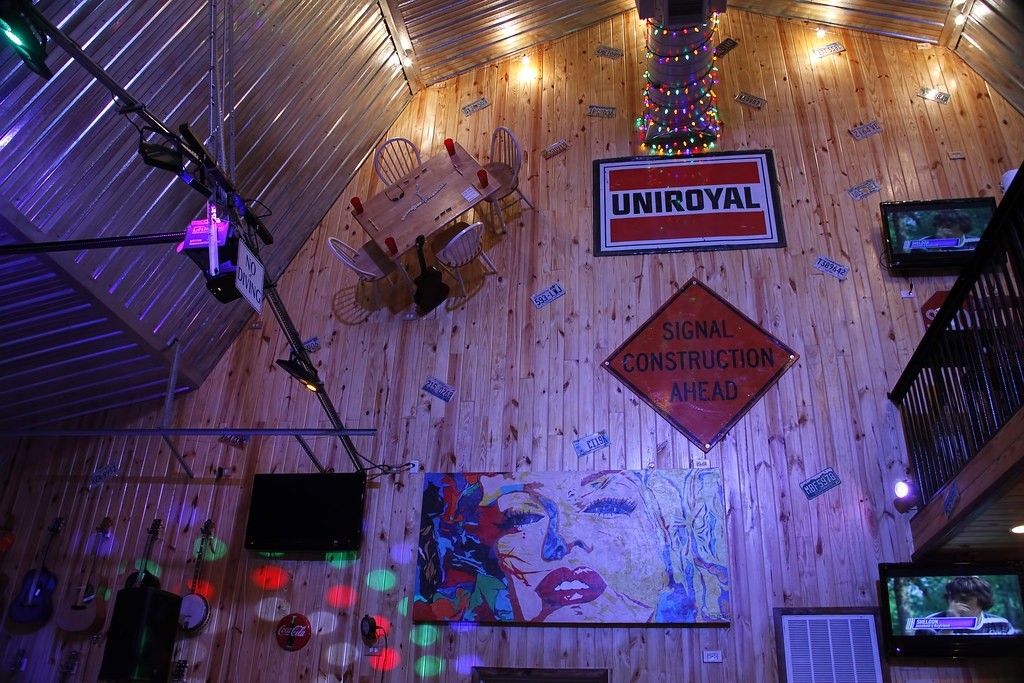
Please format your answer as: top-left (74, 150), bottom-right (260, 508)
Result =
top-left (286, 615), bottom-right (298, 648)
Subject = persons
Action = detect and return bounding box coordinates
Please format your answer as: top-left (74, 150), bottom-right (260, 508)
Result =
top-left (925, 577), bottom-right (1016, 636)
top-left (911, 209), bottom-right (981, 252)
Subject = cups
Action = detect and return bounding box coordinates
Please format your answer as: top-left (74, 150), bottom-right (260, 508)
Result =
top-left (350, 197), bottom-right (364, 213)
top-left (444, 138), bottom-right (455, 155)
top-left (477, 170), bottom-right (488, 187)
top-left (385, 237), bottom-right (398, 254)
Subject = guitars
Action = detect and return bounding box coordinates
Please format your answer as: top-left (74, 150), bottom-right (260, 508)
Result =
top-left (57, 515), bottom-right (114, 639)
top-left (124, 518), bottom-right (163, 589)
top-left (12, 522), bottom-right (57, 623)
top-left (181, 519), bottom-right (213, 635)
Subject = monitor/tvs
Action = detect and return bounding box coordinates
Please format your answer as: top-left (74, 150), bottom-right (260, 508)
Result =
top-left (877, 559), bottom-right (1024, 669)
top-left (244, 472), bottom-right (362, 555)
top-left (879, 195), bottom-right (1009, 278)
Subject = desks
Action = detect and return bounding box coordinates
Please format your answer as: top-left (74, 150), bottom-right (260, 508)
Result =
top-left (352, 141), bottom-right (506, 299)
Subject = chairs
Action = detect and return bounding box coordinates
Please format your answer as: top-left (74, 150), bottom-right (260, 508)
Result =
top-left (375, 138), bottom-right (421, 189)
top-left (327, 237), bottom-right (416, 292)
top-left (480, 125), bottom-right (535, 235)
top-left (430, 222), bottom-right (497, 297)
